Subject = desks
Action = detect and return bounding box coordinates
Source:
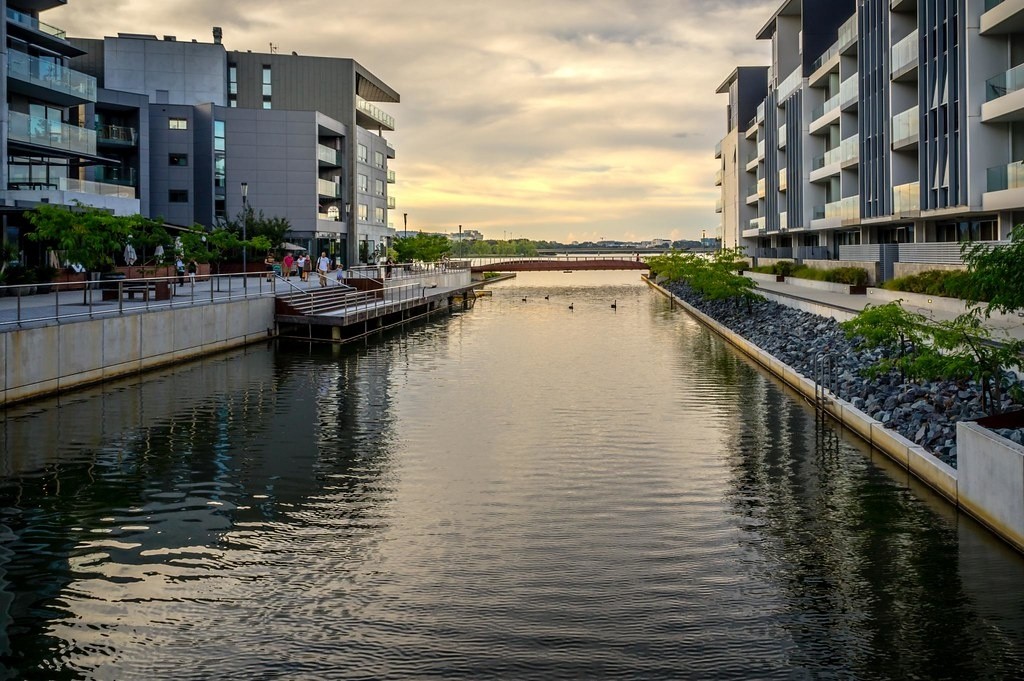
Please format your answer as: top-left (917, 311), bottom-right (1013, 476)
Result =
top-left (102, 275), bottom-right (171, 300)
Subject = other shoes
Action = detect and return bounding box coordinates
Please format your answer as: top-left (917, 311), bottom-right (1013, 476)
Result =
top-left (179, 284), bottom-right (183, 287)
top-left (190, 285), bottom-right (195, 287)
top-left (305, 280), bottom-right (308, 282)
top-left (267, 278), bottom-right (271, 282)
top-left (301, 279), bottom-right (302, 281)
top-left (338, 284), bottom-right (340, 286)
top-left (287, 279), bottom-right (291, 281)
top-left (321, 285), bottom-right (325, 288)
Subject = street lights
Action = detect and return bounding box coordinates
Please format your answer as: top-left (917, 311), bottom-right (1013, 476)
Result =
top-left (458, 225), bottom-right (462, 260)
top-left (240, 182), bottom-right (249, 287)
top-left (404, 213), bottom-right (407, 246)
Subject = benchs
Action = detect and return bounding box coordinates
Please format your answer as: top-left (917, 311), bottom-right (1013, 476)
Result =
top-left (103, 289), bottom-right (149, 302)
top-left (127, 287), bottom-right (171, 295)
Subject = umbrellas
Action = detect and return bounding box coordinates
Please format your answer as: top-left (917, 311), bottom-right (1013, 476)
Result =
top-left (278, 242), bottom-right (307, 251)
top-left (123, 235), bottom-right (137, 278)
top-left (175, 237), bottom-right (185, 260)
top-left (154, 245), bottom-right (165, 264)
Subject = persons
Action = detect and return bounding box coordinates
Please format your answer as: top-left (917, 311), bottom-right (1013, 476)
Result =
top-left (335, 264), bottom-right (344, 285)
top-left (175, 256), bottom-right (186, 287)
top-left (441, 254), bottom-right (448, 274)
top-left (316, 252), bottom-right (330, 288)
top-left (636, 254), bottom-right (639, 261)
top-left (385, 257), bottom-right (395, 280)
top-left (188, 258), bottom-right (199, 287)
top-left (264, 252), bottom-right (313, 282)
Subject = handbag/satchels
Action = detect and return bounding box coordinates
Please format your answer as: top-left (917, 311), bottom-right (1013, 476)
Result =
top-left (179, 266), bottom-right (185, 272)
top-left (317, 263), bottom-right (320, 273)
top-left (302, 268), bottom-right (305, 279)
